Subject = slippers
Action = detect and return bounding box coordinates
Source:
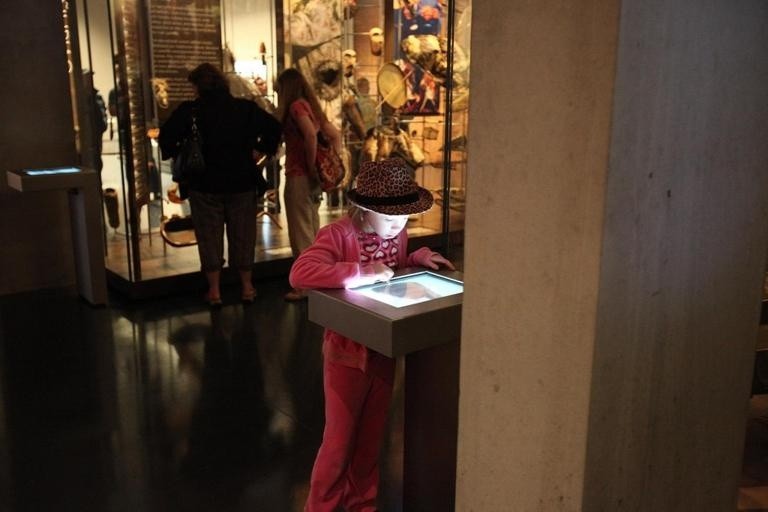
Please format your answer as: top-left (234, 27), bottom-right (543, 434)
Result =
top-left (206, 295), bottom-right (222, 304)
top-left (243, 288), bottom-right (258, 303)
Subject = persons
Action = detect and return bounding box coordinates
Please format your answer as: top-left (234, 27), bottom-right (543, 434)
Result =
top-left (287, 156), bottom-right (456, 512)
top-left (254, 77), bottom-right (280, 214)
top-left (83, 67), bottom-right (107, 173)
top-left (108, 53), bottom-right (162, 202)
top-left (353, 78), bottom-right (379, 182)
top-left (273, 67), bottom-right (341, 303)
top-left (319, 87), bottom-right (368, 218)
top-left (157, 63), bottom-right (284, 305)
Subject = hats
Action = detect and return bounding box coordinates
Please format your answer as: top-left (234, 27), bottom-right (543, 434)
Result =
top-left (345, 157), bottom-right (433, 216)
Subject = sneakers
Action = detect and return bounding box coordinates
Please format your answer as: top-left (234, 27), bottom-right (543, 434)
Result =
top-left (285, 287), bottom-right (310, 300)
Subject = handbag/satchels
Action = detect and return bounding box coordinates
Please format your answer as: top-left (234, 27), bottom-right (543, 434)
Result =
top-left (166, 99), bottom-right (209, 187)
top-left (285, 99), bottom-right (348, 193)
